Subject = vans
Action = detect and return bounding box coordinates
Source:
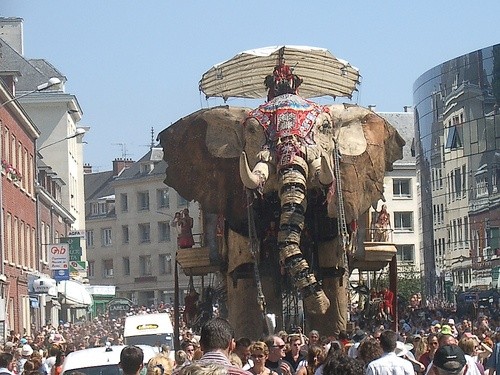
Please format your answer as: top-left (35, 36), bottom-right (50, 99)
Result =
top-left (124, 313), bottom-right (174, 351)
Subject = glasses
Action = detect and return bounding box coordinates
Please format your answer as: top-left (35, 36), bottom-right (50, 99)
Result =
top-left (279, 345), bottom-right (285, 349)
top-left (294, 344), bottom-right (301, 346)
top-left (448, 324), bottom-right (453, 325)
top-left (188, 350), bottom-right (193, 351)
top-left (430, 342), bottom-right (437, 345)
top-left (312, 336), bottom-right (317, 338)
top-left (251, 355), bottom-right (263, 358)
top-left (415, 338), bottom-right (420, 339)
top-left (480, 308), bottom-right (484, 309)
top-left (375, 337), bottom-right (380, 340)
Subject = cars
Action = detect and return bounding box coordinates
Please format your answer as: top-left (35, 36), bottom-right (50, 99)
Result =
top-left (63, 344), bottom-right (159, 375)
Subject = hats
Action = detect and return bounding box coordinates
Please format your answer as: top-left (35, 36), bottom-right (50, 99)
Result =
top-left (480, 306), bottom-right (484, 308)
top-left (433, 344), bottom-right (466, 370)
top-left (434, 324), bottom-right (441, 328)
top-left (414, 335), bottom-right (421, 339)
top-left (448, 318), bottom-right (454, 324)
top-left (21, 344), bottom-right (33, 356)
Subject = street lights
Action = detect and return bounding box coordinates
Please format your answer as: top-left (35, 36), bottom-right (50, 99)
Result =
top-left (1, 75), bottom-right (61, 108)
top-left (33, 126), bottom-right (86, 269)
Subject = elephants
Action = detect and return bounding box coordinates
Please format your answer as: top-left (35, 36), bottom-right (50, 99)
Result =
top-left (156, 94), bottom-right (406, 351)
